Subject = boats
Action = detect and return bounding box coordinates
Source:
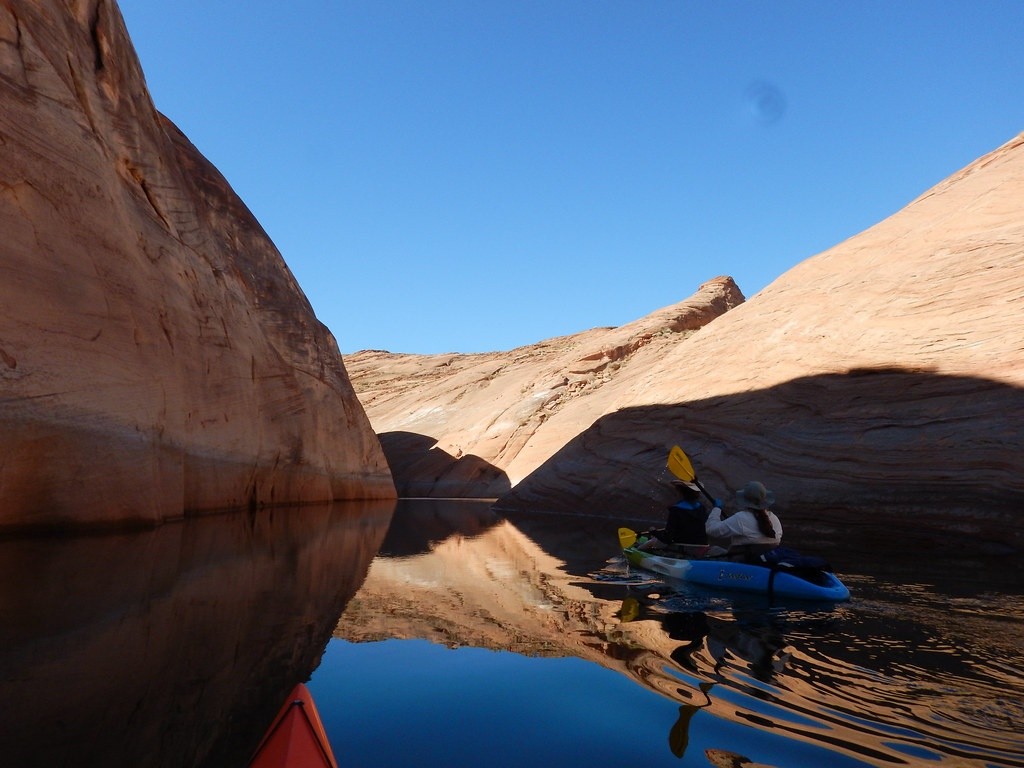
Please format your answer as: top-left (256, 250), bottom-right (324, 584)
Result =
top-left (623, 538), bottom-right (851, 602)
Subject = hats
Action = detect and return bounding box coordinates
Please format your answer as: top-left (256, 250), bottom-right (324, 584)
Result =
top-left (736, 481), bottom-right (776, 509)
top-left (670, 476), bottom-right (704, 491)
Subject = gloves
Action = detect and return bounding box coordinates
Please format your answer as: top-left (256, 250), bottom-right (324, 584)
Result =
top-left (713, 498), bottom-right (724, 508)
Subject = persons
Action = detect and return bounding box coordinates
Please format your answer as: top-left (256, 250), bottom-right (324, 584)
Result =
top-left (706, 481), bottom-right (783, 561)
top-left (638, 479), bottom-right (708, 559)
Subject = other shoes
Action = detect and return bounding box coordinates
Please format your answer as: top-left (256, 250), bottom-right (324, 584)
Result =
top-left (648, 526), bottom-right (657, 539)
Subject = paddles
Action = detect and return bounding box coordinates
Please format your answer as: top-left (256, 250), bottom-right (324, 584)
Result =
top-left (619, 526), bottom-right (667, 547)
top-left (666, 446), bottom-right (720, 507)
